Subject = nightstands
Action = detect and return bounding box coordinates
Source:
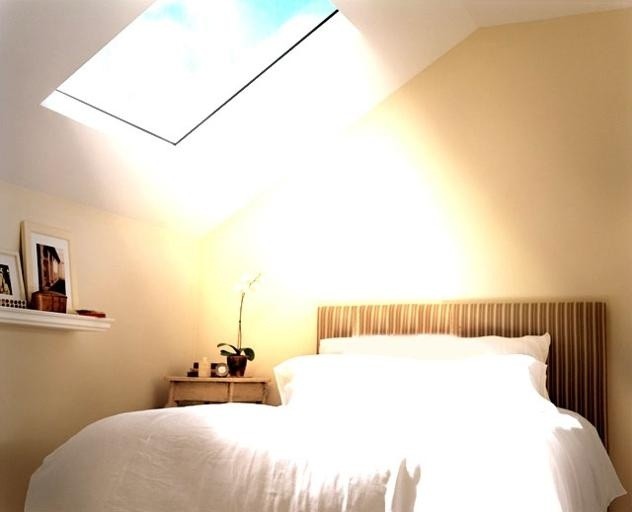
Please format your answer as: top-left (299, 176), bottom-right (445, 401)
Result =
top-left (163, 376), bottom-right (272, 409)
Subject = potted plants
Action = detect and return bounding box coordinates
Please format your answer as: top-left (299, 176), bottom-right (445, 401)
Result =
top-left (216, 340), bottom-right (255, 377)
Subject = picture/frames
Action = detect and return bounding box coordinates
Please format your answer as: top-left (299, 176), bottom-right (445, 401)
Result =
top-left (0, 219), bottom-right (79, 314)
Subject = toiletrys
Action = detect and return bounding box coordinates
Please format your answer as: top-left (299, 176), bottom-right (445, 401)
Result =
top-left (198, 356), bottom-right (211, 377)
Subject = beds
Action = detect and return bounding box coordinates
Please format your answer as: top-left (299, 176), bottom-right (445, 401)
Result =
top-left (25, 298), bottom-right (608, 512)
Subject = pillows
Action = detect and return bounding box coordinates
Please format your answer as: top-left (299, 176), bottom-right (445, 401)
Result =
top-left (319, 332), bottom-right (555, 368)
